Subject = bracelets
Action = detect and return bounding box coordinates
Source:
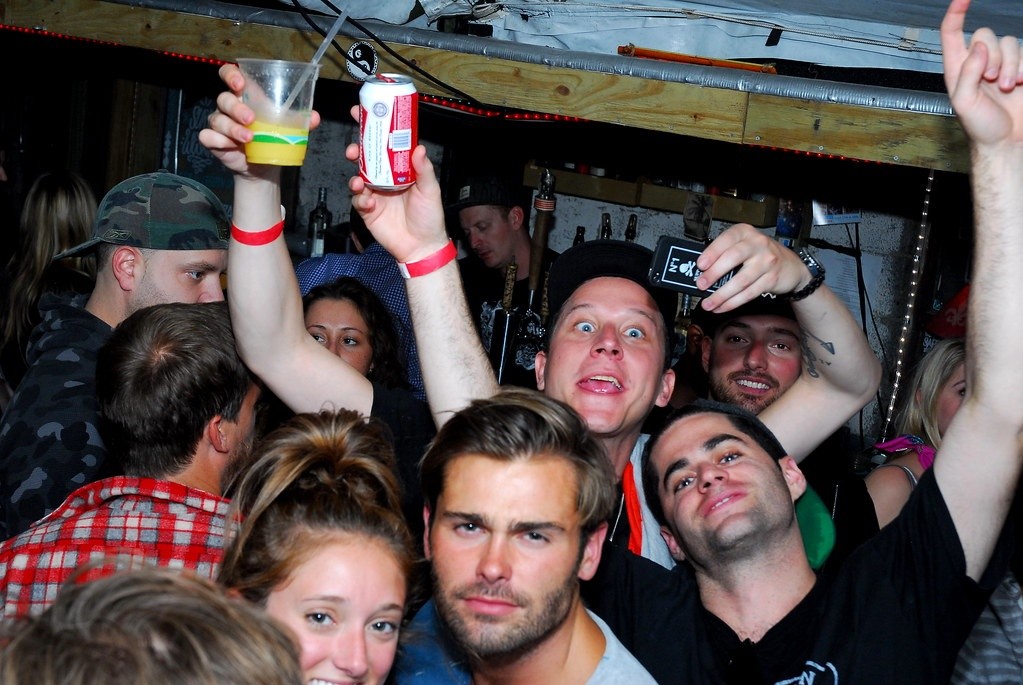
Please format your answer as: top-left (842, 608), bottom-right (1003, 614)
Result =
top-left (230, 201), bottom-right (287, 246)
top-left (396, 236), bottom-right (459, 279)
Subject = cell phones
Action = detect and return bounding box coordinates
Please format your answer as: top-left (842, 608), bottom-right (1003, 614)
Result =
top-left (647, 234), bottom-right (745, 298)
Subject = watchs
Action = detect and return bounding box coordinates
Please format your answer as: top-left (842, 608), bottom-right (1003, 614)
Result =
top-left (779, 244), bottom-right (827, 303)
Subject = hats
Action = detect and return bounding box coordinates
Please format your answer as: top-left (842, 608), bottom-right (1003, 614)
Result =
top-left (689, 292), bottom-right (797, 340)
top-left (52, 169), bottom-right (232, 260)
top-left (446, 162), bottom-right (530, 215)
top-left (547, 239), bottom-right (679, 335)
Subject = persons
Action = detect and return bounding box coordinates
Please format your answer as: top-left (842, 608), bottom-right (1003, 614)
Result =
top-left (9, 0), bottom-right (1023, 685)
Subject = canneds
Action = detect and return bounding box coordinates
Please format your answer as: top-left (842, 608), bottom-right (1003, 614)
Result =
top-left (359, 73), bottom-right (417, 191)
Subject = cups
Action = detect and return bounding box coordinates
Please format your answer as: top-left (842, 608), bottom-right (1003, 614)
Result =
top-left (235, 57), bottom-right (323, 166)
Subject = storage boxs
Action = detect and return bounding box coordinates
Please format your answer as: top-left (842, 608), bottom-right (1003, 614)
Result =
top-left (524, 152), bottom-right (780, 228)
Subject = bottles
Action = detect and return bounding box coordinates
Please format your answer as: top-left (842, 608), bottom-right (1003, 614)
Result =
top-left (305, 187), bottom-right (333, 259)
top-left (673, 293), bottom-right (694, 360)
